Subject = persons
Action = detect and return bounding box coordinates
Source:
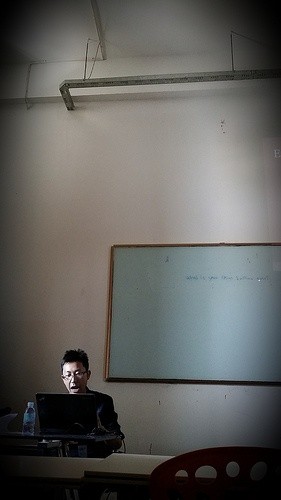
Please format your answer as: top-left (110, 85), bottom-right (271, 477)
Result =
top-left (59, 348), bottom-right (124, 458)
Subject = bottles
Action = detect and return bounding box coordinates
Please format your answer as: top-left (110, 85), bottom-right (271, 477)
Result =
top-left (22, 401), bottom-right (36, 436)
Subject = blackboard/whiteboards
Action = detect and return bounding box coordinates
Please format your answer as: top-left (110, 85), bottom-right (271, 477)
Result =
top-left (104, 243), bottom-right (281, 387)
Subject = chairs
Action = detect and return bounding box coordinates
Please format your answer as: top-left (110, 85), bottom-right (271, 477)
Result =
top-left (147, 445), bottom-right (281, 500)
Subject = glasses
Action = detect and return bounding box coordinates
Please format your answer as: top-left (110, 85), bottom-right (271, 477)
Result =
top-left (61, 371), bottom-right (88, 380)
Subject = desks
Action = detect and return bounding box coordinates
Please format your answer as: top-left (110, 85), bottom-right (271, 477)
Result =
top-left (0, 450), bottom-right (269, 500)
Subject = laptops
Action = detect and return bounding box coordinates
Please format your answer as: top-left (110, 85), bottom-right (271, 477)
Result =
top-left (35, 392), bottom-right (111, 433)
